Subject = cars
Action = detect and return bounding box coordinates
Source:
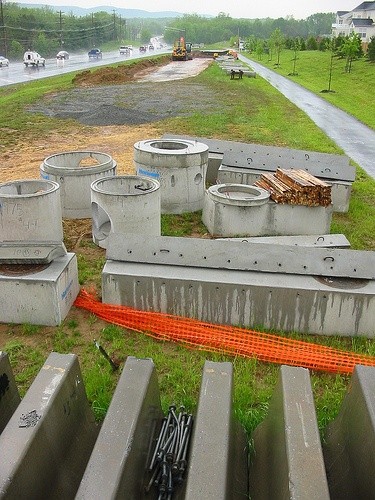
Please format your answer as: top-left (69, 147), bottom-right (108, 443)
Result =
top-left (87, 49), bottom-right (103, 57)
top-left (56, 50), bottom-right (69, 60)
top-left (139, 46), bottom-right (146, 51)
top-left (0, 56), bottom-right (10, 66)
top-left (148, 43), bottom-right (154, 49)
top-left (119, 45), bottom-right (134, 54)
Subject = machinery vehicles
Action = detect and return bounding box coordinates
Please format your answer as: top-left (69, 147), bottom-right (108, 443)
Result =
top-left (172, 36), bottom-right (193, 61)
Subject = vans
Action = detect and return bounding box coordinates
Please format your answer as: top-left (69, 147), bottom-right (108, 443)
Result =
top-left (24, 51), bottom-right (46, 66)
top-left (239, 41), bottom-right (245, 50)
